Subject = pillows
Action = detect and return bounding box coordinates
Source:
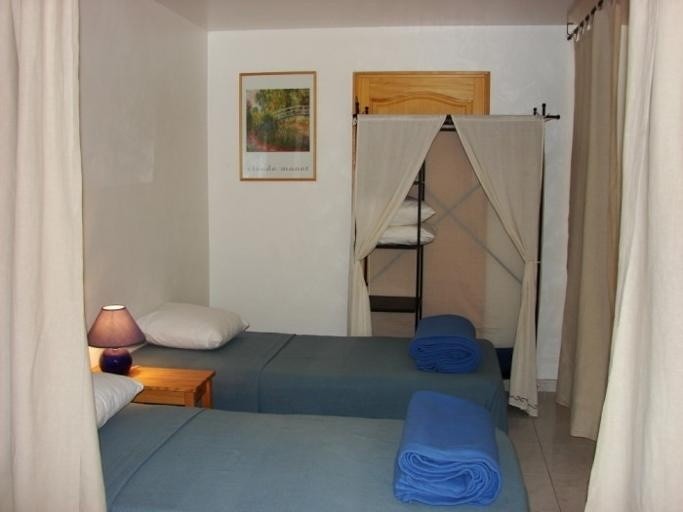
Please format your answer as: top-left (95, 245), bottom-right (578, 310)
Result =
top-left (374, 194), bottom-right (437, 246)
top-left (90, 373), bottom-right (146, 426)
top-left (137, 300), bottom-right (251, 351)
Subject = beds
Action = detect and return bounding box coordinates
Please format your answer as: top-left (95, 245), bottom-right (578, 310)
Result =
top-left (98, 402), bottom-right (530, 512)
top-left (89, 333), bottom-right (511, 433)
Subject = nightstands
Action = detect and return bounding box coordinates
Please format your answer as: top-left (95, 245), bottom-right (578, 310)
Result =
top-left (92, 365), bottom-right (217, 410)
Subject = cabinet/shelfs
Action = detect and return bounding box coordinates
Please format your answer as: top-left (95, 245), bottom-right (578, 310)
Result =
top-left (361, 160), bottom-right (425, 335)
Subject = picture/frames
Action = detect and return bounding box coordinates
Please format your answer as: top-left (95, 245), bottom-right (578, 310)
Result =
top-left (239, 70), bottom-right (318, 183)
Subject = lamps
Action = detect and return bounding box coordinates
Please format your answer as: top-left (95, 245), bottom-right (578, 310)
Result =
top-left (86, 304), bottom-right (147, 374)
